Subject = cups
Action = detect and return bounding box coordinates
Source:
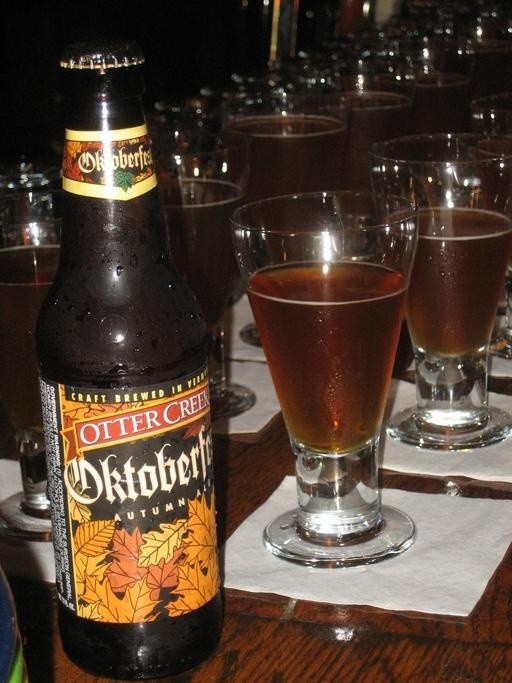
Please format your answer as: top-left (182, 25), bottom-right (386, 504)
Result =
top-left (0, 1), bottom-right (512, 574)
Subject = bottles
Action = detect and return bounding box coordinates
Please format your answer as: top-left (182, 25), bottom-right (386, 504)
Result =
top-left (44, 44), bottom-right (225, 680)
